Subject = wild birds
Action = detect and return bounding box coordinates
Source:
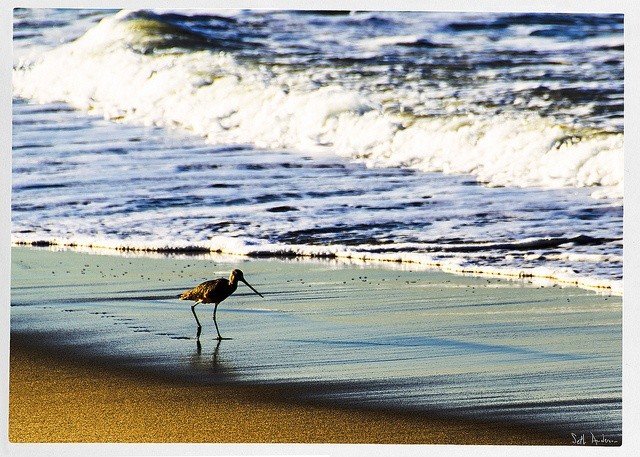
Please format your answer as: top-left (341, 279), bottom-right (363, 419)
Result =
top-left (179, 269), bottom-right (265, 340)
